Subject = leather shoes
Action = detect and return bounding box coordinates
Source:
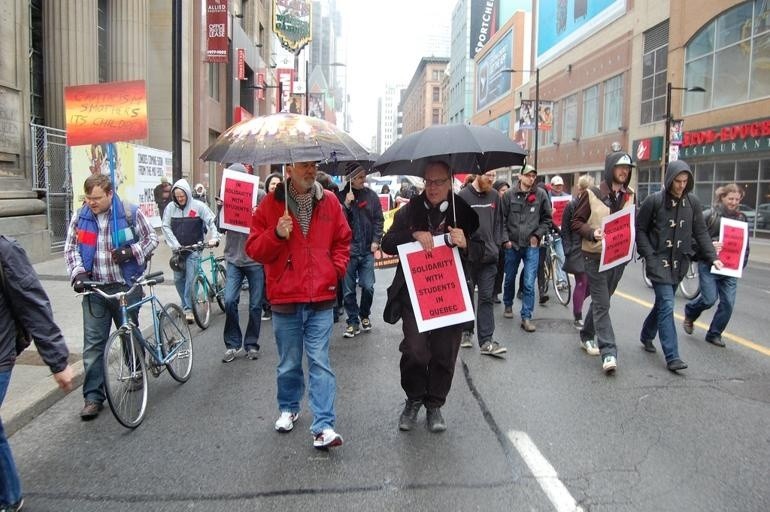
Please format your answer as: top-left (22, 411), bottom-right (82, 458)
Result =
top-left (667, 358), bottom-right (687, 370)
top-left (640, 337), bottom-right (657, 351)
top-left (684, 316), bottom-right (693, 334)
top-left (706, 334), bottom-right (725, 346)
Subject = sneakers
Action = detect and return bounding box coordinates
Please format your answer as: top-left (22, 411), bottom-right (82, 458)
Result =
top-left (127, 371), bottom-right (143, 390)
top-left (342, 318), bottom-right (372, 337)
top-left (400, 399), bottom-right (446, 432)
top-left (313, 429), bottom-right (343, 446)
top-left (462, 290), bottom-right (549, 354)
top-left (223, 347), bottom-right (259, 362)
top-left (573, 318), bottom-right (617, 371)
top-left (80, 401), bottom-right (104, 418)
top-left (275, 412), bottom-right (299, 431)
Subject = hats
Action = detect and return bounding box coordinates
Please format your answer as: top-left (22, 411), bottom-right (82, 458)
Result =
top-left (520, 165), bottom-right (537, 174)
top-left (551, 176), bottom-right (564, 185)
top-left (616, 154), bottom-right (635, 166)
top-left (344, 161), bottom-right (364, 182)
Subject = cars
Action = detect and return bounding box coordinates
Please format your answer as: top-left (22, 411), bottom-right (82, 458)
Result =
top-left (730, 203), bottom-right (763, 229)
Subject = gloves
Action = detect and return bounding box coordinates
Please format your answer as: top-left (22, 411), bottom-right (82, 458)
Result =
top-left (74, 274), bottom-right (91, 293)
top-left (111, 247), bottom-right (133, 263)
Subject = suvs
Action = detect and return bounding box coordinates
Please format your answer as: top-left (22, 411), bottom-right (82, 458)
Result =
top-left (756, 202), bottom-right (770, 229)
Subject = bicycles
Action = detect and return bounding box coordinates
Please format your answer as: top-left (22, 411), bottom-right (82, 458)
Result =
top-left (172, 241), bottom-right (227, 330)
top-left (537, 228), bottom-right (572, 310)
top-left (73, 270), bottom-right (195, 429)
top-left (640, 244), bottom-right (701, 299)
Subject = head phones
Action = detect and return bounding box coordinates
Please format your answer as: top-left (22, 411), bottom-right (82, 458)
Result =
top-left (424, 185), bottom-right (450, 213)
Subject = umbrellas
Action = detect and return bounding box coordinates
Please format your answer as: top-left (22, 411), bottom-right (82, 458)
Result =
top-left (318, 152), bottom-right (381, 204)
top-left (371, 124), bottom-right (528, 249)
top-left (197, 112), bottom-right (371, 241)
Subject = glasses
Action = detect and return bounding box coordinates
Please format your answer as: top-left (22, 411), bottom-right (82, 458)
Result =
top-left (423, 178), bottom-right (448, 185)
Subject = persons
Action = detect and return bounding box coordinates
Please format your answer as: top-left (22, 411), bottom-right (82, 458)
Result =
top-left (0, 234), bottom-right (75, 512)
top-left (246, 162), bottom-right (351, 447)
top-left (214, 163), bottom-right (284, 363)
top-left (381, 178), bottom-right (418, 210)
top-left (381, 158), bottom-right (481, 432)
top-left (682, 182), bottom-right (750, 348)
top-left (636, 159), bottom-right (724, 373)
top-left (540, 105), bottom-right (552, 124)
top-left (316, 163), bottom-right (384, 336)
top-left (154, 176), bottom-right (218, 323)
top-left (578, 151), bottom-right (639, 373)
top-left (561, 173), bottom-right (596, 331)
top-left (63, 172), bottom-right (160, 419)
top-left (457, 163), bottom-right (573, 354)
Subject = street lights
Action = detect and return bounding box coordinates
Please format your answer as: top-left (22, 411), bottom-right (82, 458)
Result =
top-left (500, 67), bottom-right (539, 176)
top-left (662, 80), bottom-right (707, 193)
top-left (247, 81), bottom-right (283, 114)
top-left (305, 59), bottom-right (346, 118)
